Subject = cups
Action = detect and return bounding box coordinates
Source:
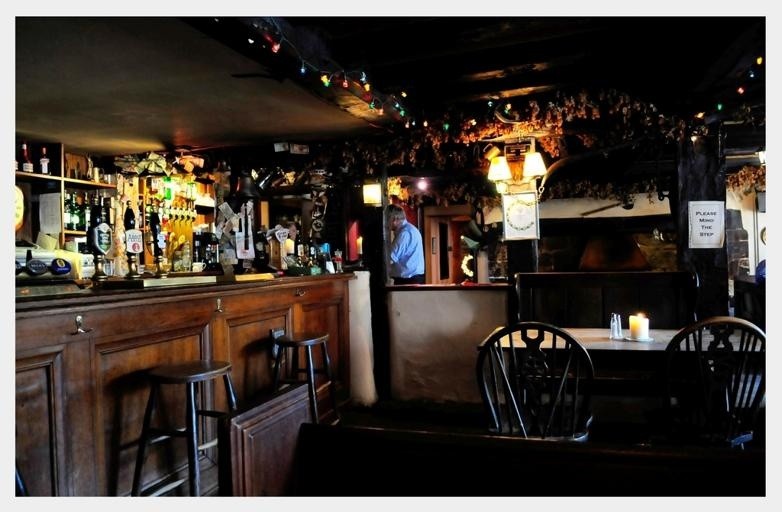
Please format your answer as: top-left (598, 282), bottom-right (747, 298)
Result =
top-left (192, 262), bottom-right (207, 272)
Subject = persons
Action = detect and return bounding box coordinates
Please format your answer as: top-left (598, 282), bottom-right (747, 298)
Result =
top-left (283, 222), bottom-right (297, 241)
top-left (386, 204), bottom-right (425, 285)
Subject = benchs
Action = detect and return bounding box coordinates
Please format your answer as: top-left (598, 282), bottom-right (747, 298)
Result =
top-left (511, 271), bottom-right (705, 429)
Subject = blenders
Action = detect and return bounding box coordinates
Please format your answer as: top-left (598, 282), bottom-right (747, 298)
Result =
top-left (193, 232), bottom-right (223, 271)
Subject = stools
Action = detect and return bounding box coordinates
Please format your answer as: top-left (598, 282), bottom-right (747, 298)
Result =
top-left (131, 330), bottom-right (331, 496)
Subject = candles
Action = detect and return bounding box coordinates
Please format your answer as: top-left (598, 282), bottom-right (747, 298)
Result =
top-left (629, 311), bottom-right (650, 340)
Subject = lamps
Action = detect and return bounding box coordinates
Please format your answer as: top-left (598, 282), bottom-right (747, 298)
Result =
top-left (362, 182), bottom-right (393, 209)
top-left (490, 152), bottom-right (547, 196)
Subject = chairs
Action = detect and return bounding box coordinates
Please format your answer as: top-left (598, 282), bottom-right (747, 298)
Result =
top-left (473, 320), bottom-right (767, 448)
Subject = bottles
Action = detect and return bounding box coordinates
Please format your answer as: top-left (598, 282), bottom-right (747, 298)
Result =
top-left (64, 189), bottom-right (108, 231)
top-left (15, 143), bottom-right (52, 176)
top-left (609, 312), bottom-right (623, 339)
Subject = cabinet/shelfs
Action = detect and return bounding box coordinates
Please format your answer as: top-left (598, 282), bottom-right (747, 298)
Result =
top-left (16, 136), bottom-right (120, 252)
